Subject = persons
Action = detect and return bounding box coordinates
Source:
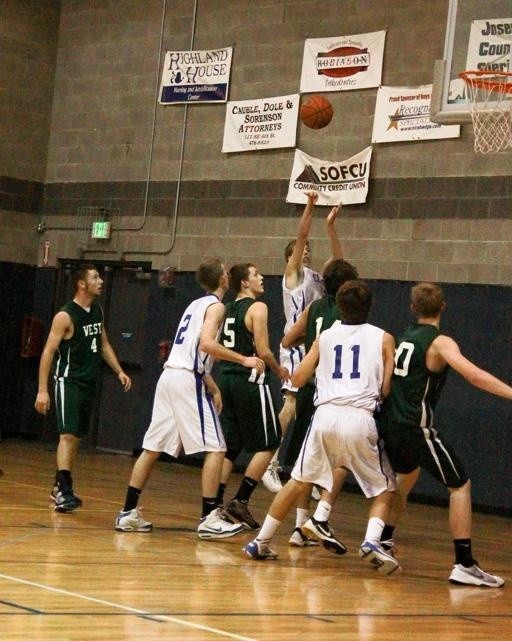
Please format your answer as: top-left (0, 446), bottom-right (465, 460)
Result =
top-left (280, 259), bottom-right (358, 555)
top-left (32, 262), bottom-right (131, 514)
top-left (242, 280), bottom-right (403, 580)
top-left (115, 258), bottom-right (265, 543)
top-left (378, 283), bottom-right (512, 588)
top-left (214, 263), bottom-right (292, 533)
top-left (223, 192), bottom-right (344, 501)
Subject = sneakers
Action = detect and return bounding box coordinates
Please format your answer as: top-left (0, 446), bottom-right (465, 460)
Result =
top-left (217, 503), bottom-right (241, 527)
top-left (300, 516), bottom-right (349, 555)
top-left (241, 538), bottom-right (279, 561)
top-left (225, 499), bottom-right (261, 534)
top-left (70, 493), bottom-right (82, 508)
top-left (358, 539), bottom-right (402, 579)
top-left (196, 508), bottom-right (242, 539)
top-left (49, 489), bottom-right (76, 511)
top-left (290, 527), bottom-right (320, 547)
top-left (448, 562), bottom-right (505, 589)
top-left (114, 507), bottom-right (153, 533)
top-left (380, 538), bottom-right (404, 563)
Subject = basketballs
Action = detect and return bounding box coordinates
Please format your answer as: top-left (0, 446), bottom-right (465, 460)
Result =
top-left (299, 94), bottom-right (335, 129)
